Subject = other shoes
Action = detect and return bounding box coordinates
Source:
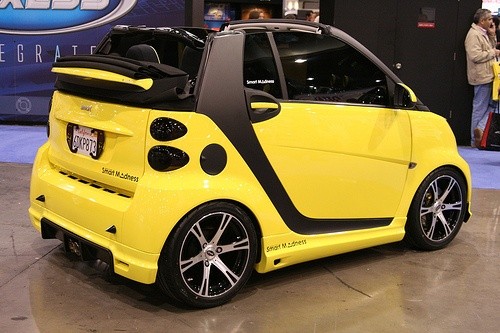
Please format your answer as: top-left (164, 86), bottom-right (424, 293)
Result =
top-left (474, 128), bottom-right (483, 148)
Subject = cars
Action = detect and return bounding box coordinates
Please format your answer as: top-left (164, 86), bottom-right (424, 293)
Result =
top-left (28, 18), bottom-right (472, 310)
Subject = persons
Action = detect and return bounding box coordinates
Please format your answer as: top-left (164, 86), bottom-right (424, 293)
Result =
top-left (464, 9), bottom-right (500, 147)
